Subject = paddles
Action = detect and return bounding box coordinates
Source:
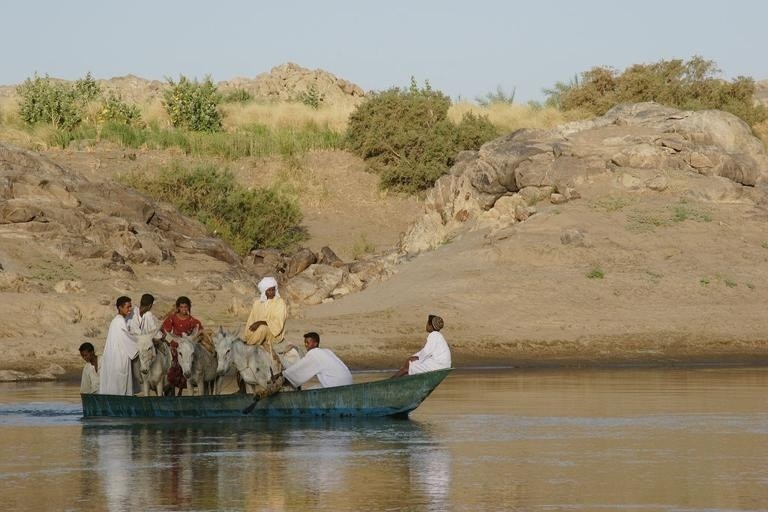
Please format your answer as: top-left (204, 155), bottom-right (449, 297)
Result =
top-left (242, 373), bottom-right (286, 415)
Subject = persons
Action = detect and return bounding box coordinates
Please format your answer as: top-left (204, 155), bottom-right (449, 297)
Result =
top-left (271, 332), bottom-right (354, 389)
top-left (127, 290), bottom-right (162, 393)
top-left (391, 315), bottom-right (450, 379)
top-left (236, 276), bottom-right (286, 394)
top-left (162, 297), bottom-right (203, 396)
top-left (79, 341), bottom-right (101, 393)
top-left (100, 296), bottom-right (139, 396)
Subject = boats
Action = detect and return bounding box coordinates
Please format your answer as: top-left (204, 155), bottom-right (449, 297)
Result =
top-left (82, 417), bottom-right (441, 452)
top-left (81, 366), bottom-right (455, 420)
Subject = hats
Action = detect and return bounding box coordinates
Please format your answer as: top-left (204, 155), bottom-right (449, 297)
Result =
top-left (431, 316), bottom-right (444, 331)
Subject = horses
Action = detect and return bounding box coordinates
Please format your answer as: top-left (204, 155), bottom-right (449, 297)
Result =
top-left (210, 324), bottom-right (301, 394)
top-left (164, 323), bottom-right (224, 396)
top-left (122, 323), bottom-right (173, 397)
